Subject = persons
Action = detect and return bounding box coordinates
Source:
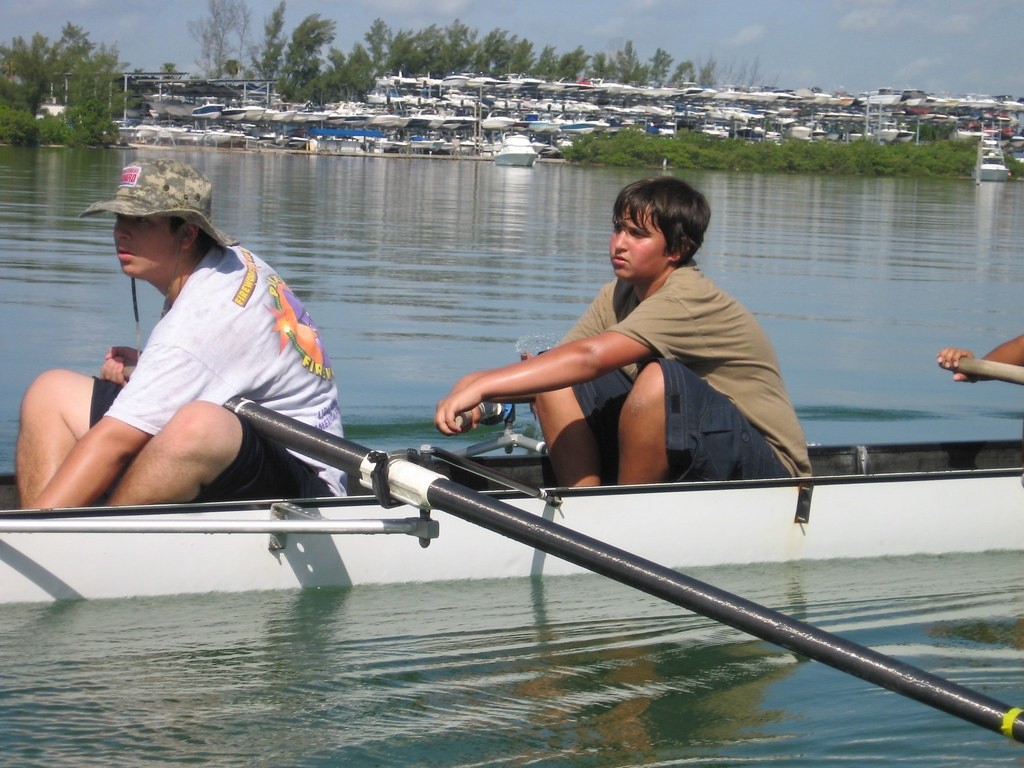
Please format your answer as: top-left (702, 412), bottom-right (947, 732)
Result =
top-left (16, 158), bottom-right (347, 511)
top-left (433, 175), bottom-right (813, 487)
top-left (936, 334), bottom-right (1024, 383)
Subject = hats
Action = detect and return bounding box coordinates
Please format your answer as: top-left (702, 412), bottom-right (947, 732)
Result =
top-left (79, 157), bottom-right (241, 247)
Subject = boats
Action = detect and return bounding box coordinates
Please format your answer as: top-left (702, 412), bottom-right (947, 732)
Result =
top-left (0, 551), bottom-right (1024, 647)
top-left (3, 434), bottom-right (1024, 606)
top-left (972, 139), bottom-right (1010, 184)
top-left (104, 67), bottom-right (1024, 169)
top-left (493, 130), bottom-right (538, 168)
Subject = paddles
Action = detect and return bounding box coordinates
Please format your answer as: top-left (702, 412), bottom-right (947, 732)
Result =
top-left (953, 353), bottom-right (1024, 389)
top-left (121, 360), bottom-right (1024, 744)
top-left (450, 349), bottom-right (535, 430)
